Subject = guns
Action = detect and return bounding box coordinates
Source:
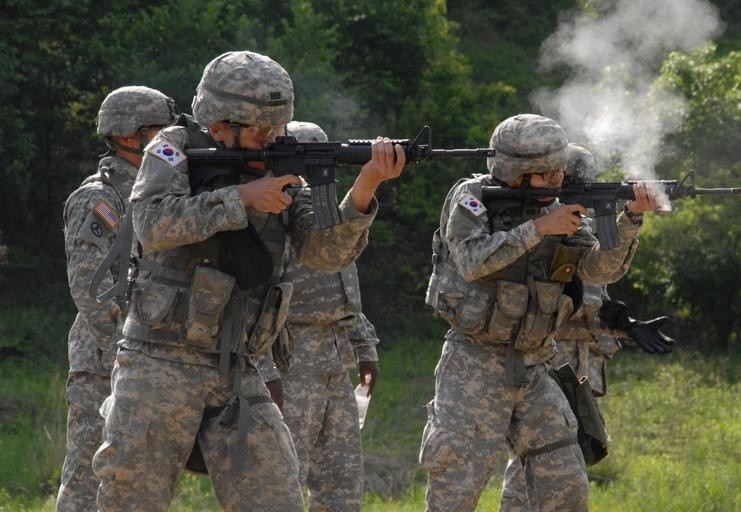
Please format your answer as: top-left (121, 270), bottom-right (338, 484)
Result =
top-left (185, 125), bottom-right (495, 231)
top-left (481, 171), bottom-right (741, 251)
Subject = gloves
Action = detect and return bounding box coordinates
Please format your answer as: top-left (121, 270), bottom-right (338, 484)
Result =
top-left (598, 298), bottom-right (632, 333)
top-left (629, 316), bottom-right (677, 357)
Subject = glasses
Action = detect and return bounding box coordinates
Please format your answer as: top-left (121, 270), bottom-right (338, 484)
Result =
top-left (224, 123), bottom-right (277, 143)
top-left (526, 171), bottom-right (563, 182)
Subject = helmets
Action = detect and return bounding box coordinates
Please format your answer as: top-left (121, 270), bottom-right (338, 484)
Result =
top-left (96, 84), bottom-right (175, 139)
top-left (487, 113), bottom-right (597, 185)
top-left (190, 50), bottom-right (294, 130)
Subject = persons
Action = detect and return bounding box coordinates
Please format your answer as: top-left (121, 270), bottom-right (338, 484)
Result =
top-left (418, 112), bottom-right (659, 511)
top-left (272, 119), bottom-right (380, 512)
top-left (499, 144), bottom-right (677, 511)
top-left (45, 85), bottom-right (179, 511)
top-left (92, 49), bottom-right (406, 512)
